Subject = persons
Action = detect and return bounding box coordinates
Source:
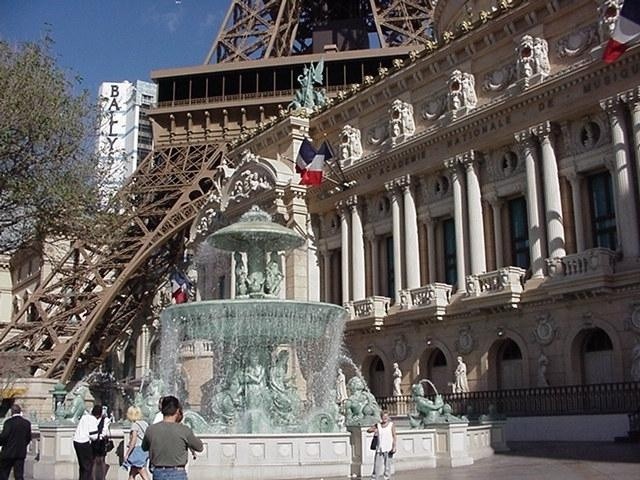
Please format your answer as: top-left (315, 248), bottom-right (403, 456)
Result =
top-left (335, 367), bottom-right (348, 402)
top-left (233, 246), bottom-right (284, 296)
top-left (122, 404), bottom-right (154, 480)
top-left (91, 403), bottom-right (113, 480)
top-left (345, 376), bottom-right (376, 421)
top-left (71, 404), bottom-right (104, 480)
top-left (140, 395), bottom-right (205, 480)
top-left (270, 347), bottom-right (296, 394)
top-left (454, 356), bottom-right (470, 393)
top-left (0, 403), bottom-right (31, 479)
top-left (391, 362), bottom-right (403, 396)
top-left (151, 397), bottom-right (165, 425)
top-left (411, 383), bottom-right (445, 425)
top-left (367, 409), bottom-right (398, 480)
top-left (238, 351), bottom-right (268, 405)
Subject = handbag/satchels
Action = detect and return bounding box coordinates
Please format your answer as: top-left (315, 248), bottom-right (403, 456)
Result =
top-left (371, 436), bottom-right (377, 450)
top-left (105, 441), bottom-right (114, 452)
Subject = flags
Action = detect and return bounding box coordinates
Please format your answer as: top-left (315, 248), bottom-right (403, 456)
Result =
top-left (295, 139), bottom-right (317, 174)
top-left (599, 0), bottom-right (640, 68)
top-left (299, 137), bottom-right (335, 187)
top-left (169, 271), bottom-right (189, 304)
